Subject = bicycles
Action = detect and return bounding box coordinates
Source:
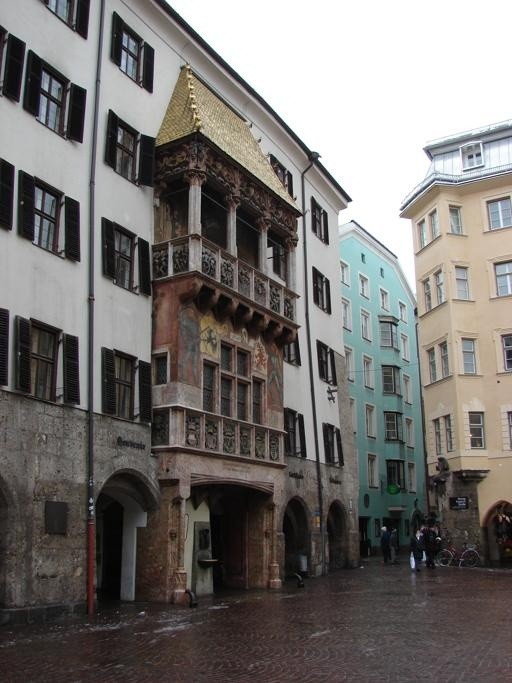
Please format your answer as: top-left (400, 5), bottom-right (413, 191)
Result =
top-left (436, 537), bottom-right (479, 568)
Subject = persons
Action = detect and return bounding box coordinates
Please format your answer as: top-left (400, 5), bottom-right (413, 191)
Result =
top-left (409, 520), bottom-right (441, 573)
top-left (380, 526), bottom-right (390, 563)
top-left (389, 526), bottom-right (400, 565)
